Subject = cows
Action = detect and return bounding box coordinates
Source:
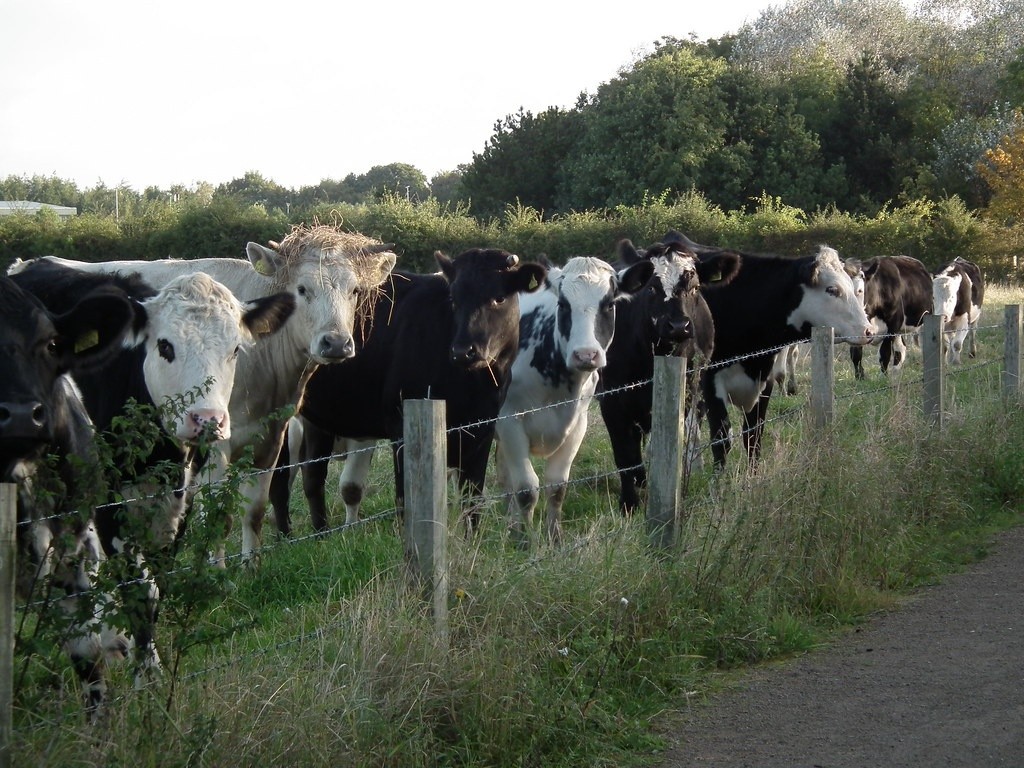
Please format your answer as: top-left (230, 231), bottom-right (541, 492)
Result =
top-left (646, 232), bottom-right (873, 479)
top-left (850, 254), bottom-right (983, 381)
top-left (0, 228), bottom-right (396, 726)
top-left (268, 229), bottom-right (740, 537)
